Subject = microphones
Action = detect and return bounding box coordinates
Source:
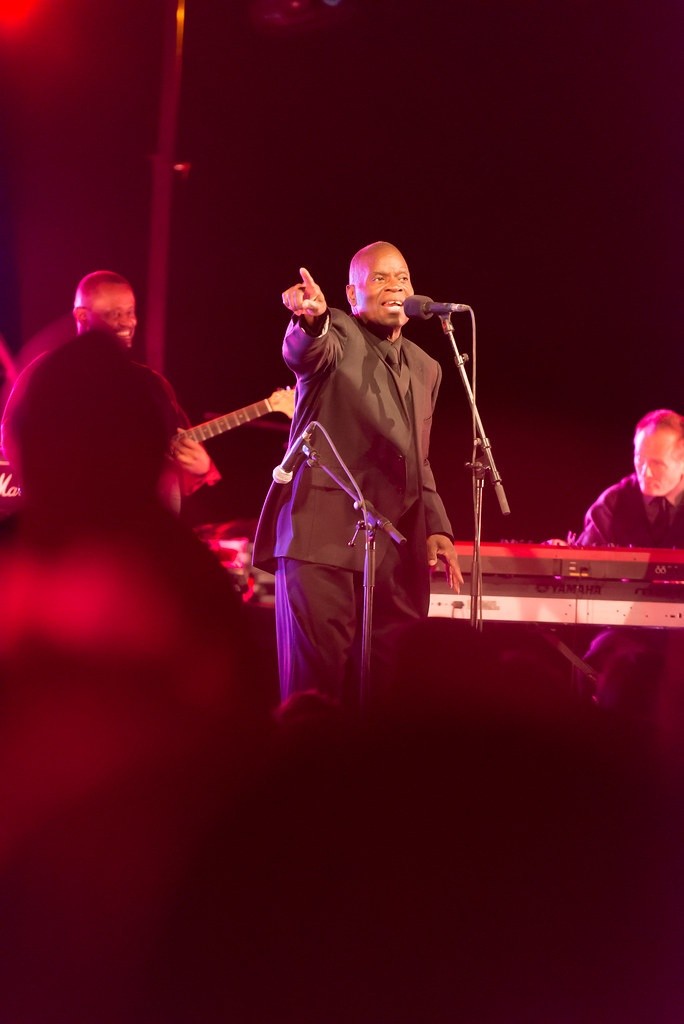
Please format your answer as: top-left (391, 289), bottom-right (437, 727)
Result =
top-left (404, 295), bottom-right (471, 320)
top-left (273, 420), bottom-right (316, 484)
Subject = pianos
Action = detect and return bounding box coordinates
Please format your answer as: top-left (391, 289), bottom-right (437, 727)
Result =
top-left (427, 538), bottom-right (684, 629)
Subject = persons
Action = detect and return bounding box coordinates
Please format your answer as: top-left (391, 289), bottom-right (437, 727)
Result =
top-left (0, 329), bottom-right (684, 1024)
top-left (251, 242), bottom-right (464, 710)
top-left (0, 270), bottom-right (224, 518)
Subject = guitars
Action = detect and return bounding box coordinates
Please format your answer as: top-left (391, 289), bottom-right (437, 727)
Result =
top-left (178, 385), bottom-right (298, 445)
